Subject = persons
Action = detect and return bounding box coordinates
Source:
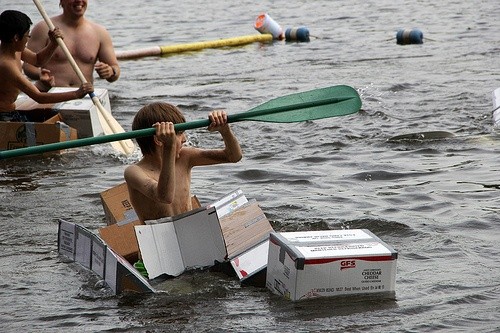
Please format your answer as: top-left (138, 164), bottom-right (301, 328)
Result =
top-left (0, 10), bottom-right (95, 122)
top-left (123, 102), bottom-right (243, 225)
top-left (23, 0), bottom-right (120, 93)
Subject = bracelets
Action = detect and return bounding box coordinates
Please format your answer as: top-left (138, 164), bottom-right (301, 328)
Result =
top-left (112, 68), bottom-right (116, 77)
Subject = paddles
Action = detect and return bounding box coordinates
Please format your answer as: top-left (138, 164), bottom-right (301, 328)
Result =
top-left (0, 84), bottom-right (362, 158)
top-left (34, 0), bottom-right (136, 155)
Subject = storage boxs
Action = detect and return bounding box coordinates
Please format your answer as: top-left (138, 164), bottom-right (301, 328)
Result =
top-left (99, 182), bottom-right (140, 225)
top-left (265, 228), bottom-right (399, 305)
top-left (97, 220), bottom-right (146, 262)
top-left (38, 87), bottom-right (111, 141)
top-left (1, 121), bottom-right (77, 156)
top-left (18, 110), bottom-right (64, 124)
